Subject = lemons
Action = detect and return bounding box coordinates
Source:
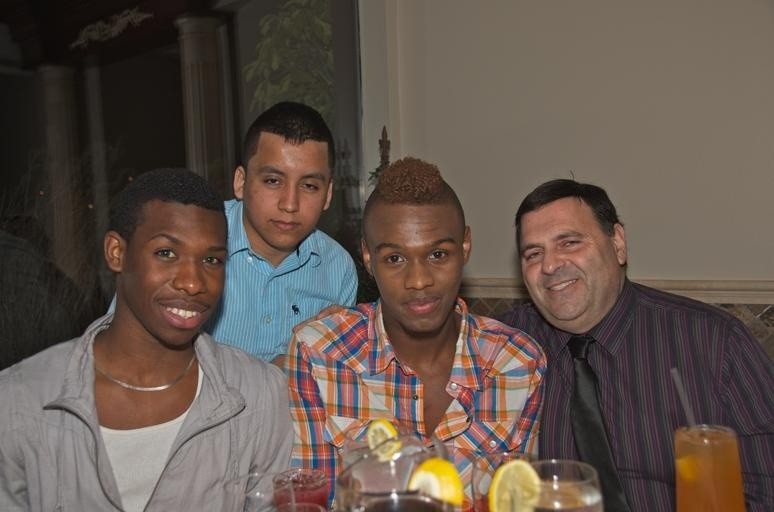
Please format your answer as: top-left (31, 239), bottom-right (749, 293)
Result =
top-left (488, 459), bottom-right (541, 512)
top-left (407, 457), bottom-right (464, 507)
top-left (367, 418), bottom-right (403, 462)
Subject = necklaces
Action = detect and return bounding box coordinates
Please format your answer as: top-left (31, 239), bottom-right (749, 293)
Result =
top-left (95, 352), bottom-right (202, 394)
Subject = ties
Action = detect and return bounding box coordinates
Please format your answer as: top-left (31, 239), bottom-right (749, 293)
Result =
top-left (567, 337), bottom-right (633, 512)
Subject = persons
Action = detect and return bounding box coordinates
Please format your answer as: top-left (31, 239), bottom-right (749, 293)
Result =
top-left (491, 167), bottom-right (774, 512)
top-left (0, 164), bottom-right (296, 511)
top-left (105, 100), bottom-right (360, 373)
top-left (281, 152), bottom-right (549, 512)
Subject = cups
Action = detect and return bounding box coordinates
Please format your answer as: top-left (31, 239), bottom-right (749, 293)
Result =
top-left (673, 425), bottom-right (746, 511)
top-left (471, 452), bottom-right (604, 512)
top-left (224, 468), bottom-right (329, 512)
top-left (344, 421), bottom-right (450, 512)
